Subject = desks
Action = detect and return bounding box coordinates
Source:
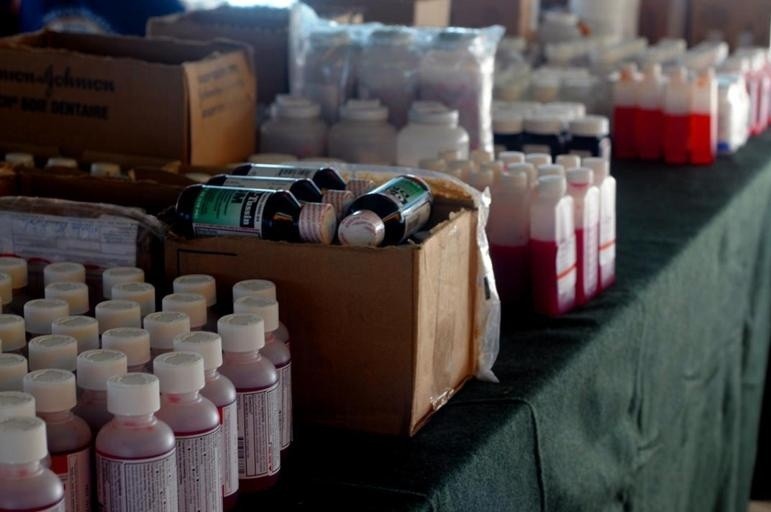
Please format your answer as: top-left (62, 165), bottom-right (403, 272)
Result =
top-left (229, 125), bottom-right (771, 512)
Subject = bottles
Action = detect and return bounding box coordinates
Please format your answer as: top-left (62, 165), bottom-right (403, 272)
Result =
top-left (494, 13), bottom-right (770, 153)
top-left (364, 25), bottom-right (417, 95)
top-left (419, 26), bottom-right (492, 170)
top-left (426, 156), bottom-right (614, 313)
top-left (523, 112), bottom-right (566, 160)
top-left (209, 173), bottom-right (351, 215)
top-left (332, 97), bottom-right (395, 166)
top-left (401, 102), bottom-right (470, 179)
top-left (234, 164), bottom-right (373, 196)
top-left (538, 102), bottom-right (584, 141)
top-left (305, 28), bottom-right (358, 93)
top-left (492, 110), bottom-right (521, 156)
top-left (177, 184), bottom-right (335, 244)
top-left (262, 96), bottom-right (328, 162)
top-left (343, 172), bottom-right (432, 246)
top-left (2, 255), bottom-right (296, 509)
top-left (570, 115), bottom-right (609, 167)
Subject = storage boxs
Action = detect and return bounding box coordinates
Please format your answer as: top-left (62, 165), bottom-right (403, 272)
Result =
top-left (176, 193), bottom-right (479, 437)
top-left (0, 0), bottom-right (771, 168)
